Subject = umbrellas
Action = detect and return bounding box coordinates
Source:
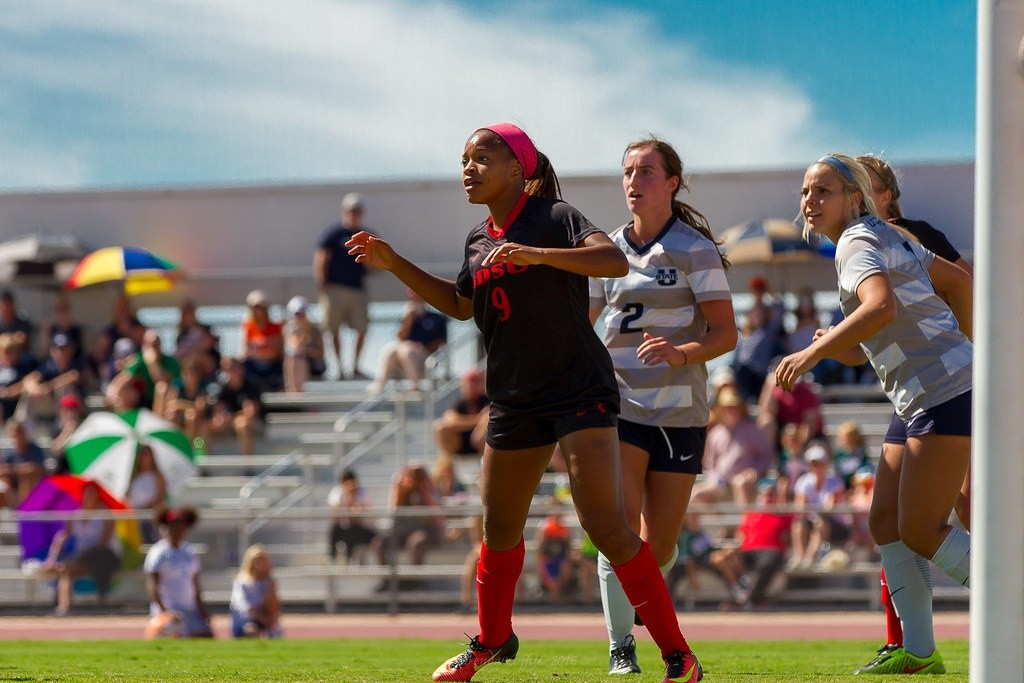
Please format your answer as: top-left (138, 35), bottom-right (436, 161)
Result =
top-left (64, 245), bottom-right (183, 294)
top-left (713, 210), bottom-right (818, 264)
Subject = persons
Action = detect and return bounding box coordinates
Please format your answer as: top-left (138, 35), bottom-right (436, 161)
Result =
top-left (0, 271), bottom-right (876, 618)
top-left (590, 128), bottom-right (738, 676)
top-left (345, 122), bottom-right (706, 683)
top-left (313, 192), bottom-right (376, 380)
top-left (772, 153), bottom-right (974, 674)
top-left (144, 507), bottom-right (212, 637)
top-left (228, 544), bottom-right (285, 639)
top-left (855, 155), bottom-right (975, 671)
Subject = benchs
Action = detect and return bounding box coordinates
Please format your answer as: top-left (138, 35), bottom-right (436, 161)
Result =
top-left (0, 302), bottom-right (970, 611)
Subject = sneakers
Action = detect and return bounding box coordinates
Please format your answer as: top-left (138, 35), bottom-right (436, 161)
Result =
top-left (431, 631), bottom-right (519, 683)
top-left (661, 651), bottom-right (703, 683)
top-left (858, 649), bottom-right (947, 675)
top-left (853, 642), bottom-right (900, 675)
top-left (608, 645), bottom-right (641, 675)
